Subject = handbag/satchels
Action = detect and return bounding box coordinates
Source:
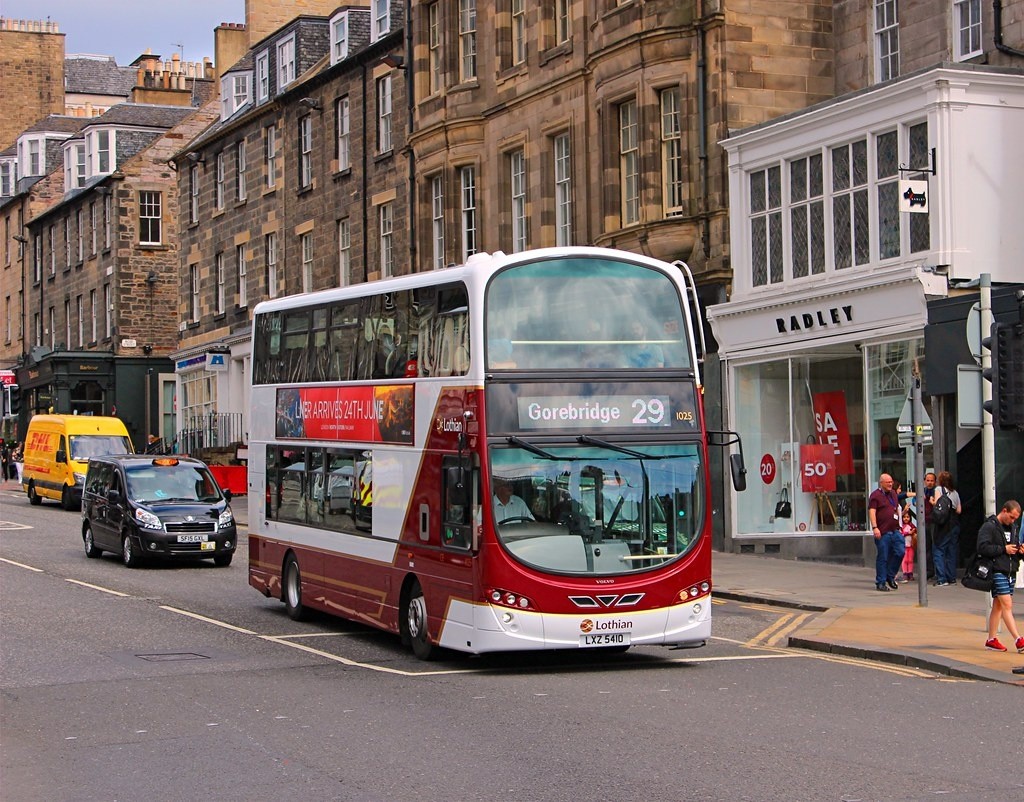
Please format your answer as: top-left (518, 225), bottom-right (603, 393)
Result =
top-left (824, 501), bottom-right (835, 525)
top-left (910, 528), bottom-right (918, 548)
top-left (774, 487), bottom-right (792, 518)
top-left (832, 475), bottom-right (845, 492)
top-left (929, 486), bottom-right (952, 526)
top-left (881, 433), bottom-right (890, 453)
top-left (960, 553), bottom-right (995, 592)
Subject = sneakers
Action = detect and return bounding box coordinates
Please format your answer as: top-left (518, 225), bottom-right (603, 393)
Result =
top-left (1015, 636), bottom-right (1024, 653)
top-left (984, 638), bottom-right (1007, 652)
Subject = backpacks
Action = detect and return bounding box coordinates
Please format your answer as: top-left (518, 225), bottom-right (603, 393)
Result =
top-left (837, 499), bottom-right (849, 516)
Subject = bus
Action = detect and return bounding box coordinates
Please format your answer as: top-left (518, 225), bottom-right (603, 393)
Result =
top-left (247, 246), bottom-right (747, 661)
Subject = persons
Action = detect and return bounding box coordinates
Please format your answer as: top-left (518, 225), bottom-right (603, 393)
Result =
top-left (620, 320), bottom-right (665, 369)
top-left (977, 499), bottom-right (1024, 653)
top-left (0, 441), bottom-right (24, 483)
top-left (148, 434), bottom-right (154, 444)
top-left (868, 471), bottom-right (961, 592)
top-left (453, 322), bottom-right (517, 373)
top-left (477, 476), bottom-right (535, 526)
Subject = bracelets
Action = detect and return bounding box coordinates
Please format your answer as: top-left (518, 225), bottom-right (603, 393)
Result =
top-left (872, 527), bottom-right (878, 529)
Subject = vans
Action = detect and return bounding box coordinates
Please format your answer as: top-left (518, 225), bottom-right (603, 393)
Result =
top-left (21, 415), bottom-right (142, 511)
top-left (80, 454), bottom-right (238, 569)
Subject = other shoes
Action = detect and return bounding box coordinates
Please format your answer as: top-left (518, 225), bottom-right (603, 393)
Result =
top-left (908, 573), bottom-right (914, 580)
top-left (950, 578), bottom-right (957, 585)
top-left (901, 574), bottom-right (909, 583)
top-left (927, 570), bottom-right (935, 578)
top-left (932, 579), bottom-right (949, 587)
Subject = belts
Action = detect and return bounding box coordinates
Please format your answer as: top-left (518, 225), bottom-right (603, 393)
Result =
top-left (887, 531), bottom-right (894, 534)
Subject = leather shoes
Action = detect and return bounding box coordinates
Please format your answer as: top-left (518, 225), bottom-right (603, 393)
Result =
top-left (875, 583), bottom-right (890, 591)
top-left (886, 574), bottom-right (898, 590)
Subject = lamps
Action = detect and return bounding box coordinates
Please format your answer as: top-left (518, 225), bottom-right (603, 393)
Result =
top-left (299, 97), bottom-right (321, 111)
top-left (380, 54), bottom-right (406, 70)
top-left (12, 234), bottom-right (28, 244)
top-left (94, 187), bottom-right (113, 199)
top-left (185, 153), bottom-right (205, 163)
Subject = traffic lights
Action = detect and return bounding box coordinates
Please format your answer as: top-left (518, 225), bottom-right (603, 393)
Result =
top-left (981, 321), bottom-right (1013, 432)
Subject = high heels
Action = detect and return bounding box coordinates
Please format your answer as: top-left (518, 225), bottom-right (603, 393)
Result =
top-left (855, 524), bottom-right (860, 531)
top-left (848, 523), bottom-right (853, 530)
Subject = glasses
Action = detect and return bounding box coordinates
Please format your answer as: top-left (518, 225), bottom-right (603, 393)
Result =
top-left (496, 482), bottom-right (516, 488)
